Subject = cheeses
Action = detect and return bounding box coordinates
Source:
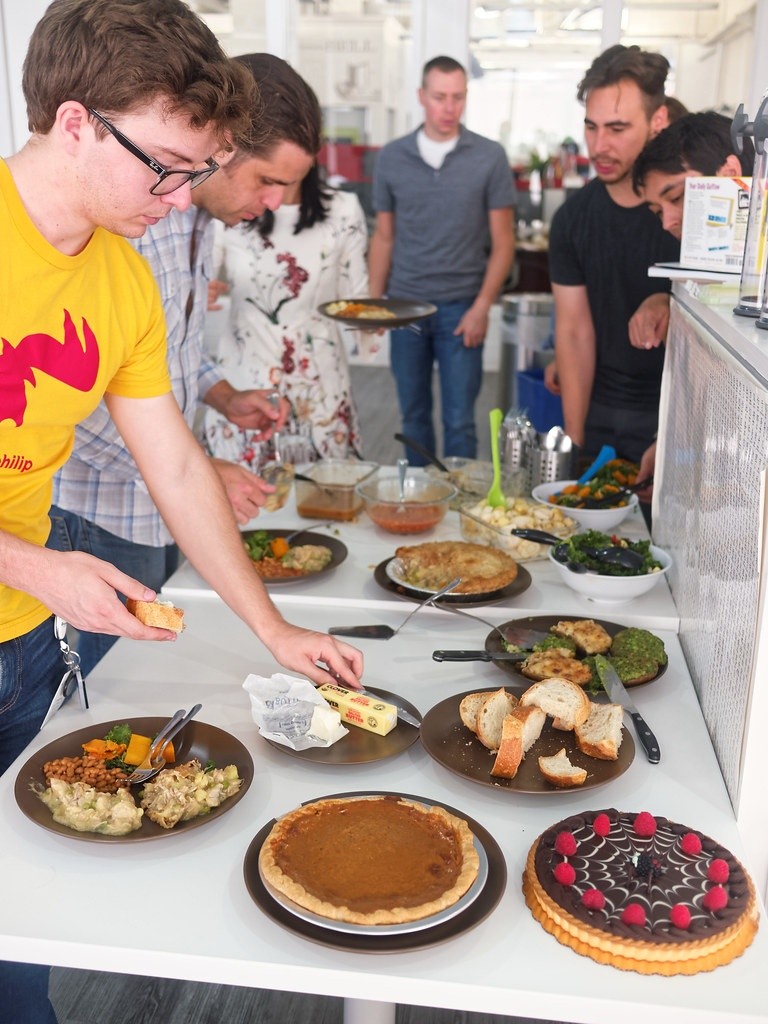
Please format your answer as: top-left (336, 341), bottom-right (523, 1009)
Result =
top-left (315, 683), bottom-right (398, 736)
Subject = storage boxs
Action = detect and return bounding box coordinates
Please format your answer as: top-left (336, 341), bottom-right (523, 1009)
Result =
top-left (425, 457), bottom-right (526, 511)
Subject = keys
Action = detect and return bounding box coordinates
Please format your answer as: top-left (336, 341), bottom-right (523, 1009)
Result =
top-left (63, 667), bottom-right (90, 711)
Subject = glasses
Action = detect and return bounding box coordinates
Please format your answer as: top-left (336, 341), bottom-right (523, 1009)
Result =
top-left (84, 106), bottom-right (220, 195)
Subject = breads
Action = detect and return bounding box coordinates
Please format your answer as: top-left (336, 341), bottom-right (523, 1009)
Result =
top-left (458, 677), bottom-right (624, 788)
top-left (125, 595), bottom-right (184, 632)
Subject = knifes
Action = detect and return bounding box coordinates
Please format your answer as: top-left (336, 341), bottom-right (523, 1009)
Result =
top-left (338, 679), bottom-right (420, 729)
top-left (431, 647), bottom-right (533, 664)
top-left (595, 653), bottom-right (660, 764)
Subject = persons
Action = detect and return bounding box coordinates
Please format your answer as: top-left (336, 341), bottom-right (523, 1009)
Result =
top-left (364, 56), bottom-right (520, 469)
top-left (547, 43), bottom-right (683, 485)
top-left (541, 95), bottom-right (691, 398)
top-left (627, 110), bottom-right (756, 506)
top-left (198, 153), bottom-right (372, 480)
top-left (1, 0), bottom-right (363, 1024)
top-left (42, 50), bottom-right (323, 677)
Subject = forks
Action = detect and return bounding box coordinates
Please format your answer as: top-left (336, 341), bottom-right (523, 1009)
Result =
top-left (503, 403), bottom-right (532, 443)
top-left (115, 707), bottom-right (186, 784)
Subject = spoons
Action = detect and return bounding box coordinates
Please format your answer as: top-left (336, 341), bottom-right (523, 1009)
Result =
top-left (540, 426), bottom-right (575, 456)
top-left (510, 526), bottom-right (644, 570)
top-left (133, 704), bottom-right (202, 785)
top-left (486, 407), bottom-right (508, 509)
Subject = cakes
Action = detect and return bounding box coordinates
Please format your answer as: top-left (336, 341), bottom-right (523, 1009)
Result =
top-left (521, 807), bottom-right (760, 977)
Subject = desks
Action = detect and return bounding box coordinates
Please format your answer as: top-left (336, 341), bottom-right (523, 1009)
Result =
top-left (0, 593), bottom-right (768, 1024)
top-left (156, 462), bottom-right (683, 638)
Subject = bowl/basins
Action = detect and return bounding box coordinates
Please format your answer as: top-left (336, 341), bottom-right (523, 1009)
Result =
top-left (356, 475), bottom-right (458, 534)
top-left (461, 500), bottom-right (581, 567)
top-left (295, 458), bottom-right (381, 520)
top-left (548, 539), bottom-right (671, 606)
top-left (428, 456), bottom-right (527, 516)
top-left (529, 481), bottom-right (639, 533)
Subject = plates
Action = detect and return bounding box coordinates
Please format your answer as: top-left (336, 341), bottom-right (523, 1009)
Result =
top-left (486, 612), bottom-right (669, 692)
top-left (263, 685), bottom-right (422, 765)
top-left (244, 791), bottom-right (508, 955)
top-left (319, 295), bottom-right (439, 328)
top-left (240, 527), bottom-right (345, 589)
top-left (14, 717), bottom-right (253, 844)
top-left (420, 685), bottom-right (634, 794)
top-left (374, 554), bottom-right (531, 607)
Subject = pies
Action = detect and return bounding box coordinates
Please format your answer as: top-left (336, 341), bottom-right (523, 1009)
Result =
top-left (386, 539), bottom-right (518, 601)
top-left (259, 795), bottom-right (481, 926)
